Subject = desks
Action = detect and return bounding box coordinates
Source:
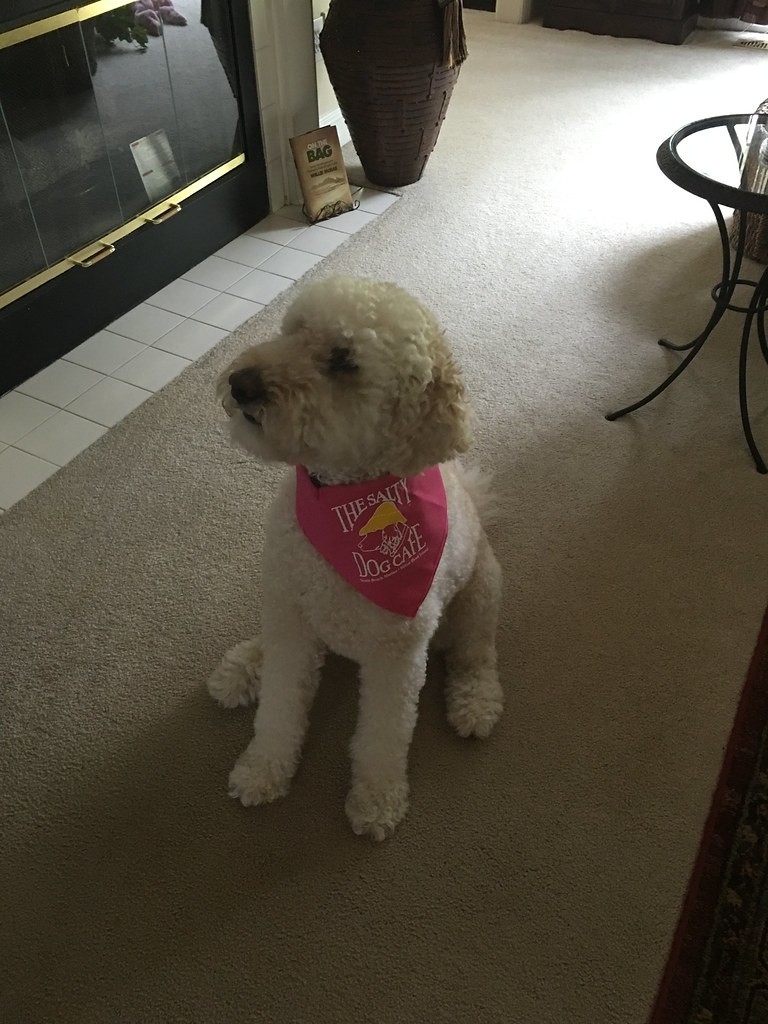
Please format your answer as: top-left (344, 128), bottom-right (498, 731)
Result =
top-left (605, 112), bottom-right (768, 474)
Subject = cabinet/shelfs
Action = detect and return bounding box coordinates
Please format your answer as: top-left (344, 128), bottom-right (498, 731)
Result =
top-left (0, 1), bottom-right (274, 396)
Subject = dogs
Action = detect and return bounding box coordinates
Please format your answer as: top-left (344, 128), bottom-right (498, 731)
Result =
top-left (205, 272), bottom-right (509, 843)
